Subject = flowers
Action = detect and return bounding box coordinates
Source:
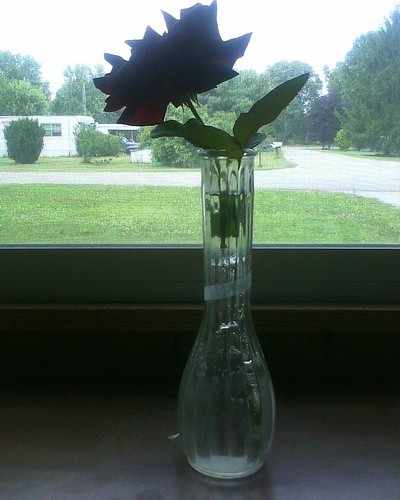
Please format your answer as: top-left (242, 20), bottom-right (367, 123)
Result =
top-left (94, 1), bottom-right (310, 386)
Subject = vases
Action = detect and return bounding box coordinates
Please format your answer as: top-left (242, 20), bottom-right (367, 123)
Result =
top-left (176, 148), bottom-right (277, 481)
